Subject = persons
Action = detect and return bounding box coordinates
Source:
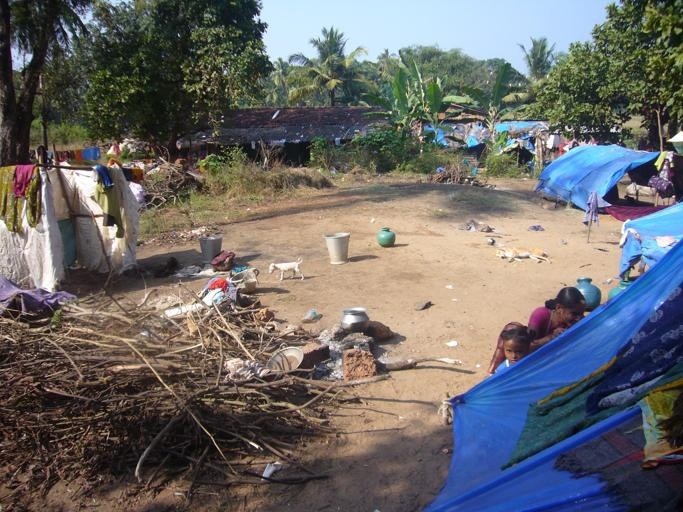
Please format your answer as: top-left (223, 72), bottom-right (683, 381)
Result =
top-left (528, 287), bottom-right (585, 354)
top-left (491, 327), bottom-right (536, 374)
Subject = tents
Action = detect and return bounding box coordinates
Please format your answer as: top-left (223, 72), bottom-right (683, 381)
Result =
top-left (534, 144), bottom-right (661, 212)
top-left (617, 200), bottom-right (682, 280)
top-left (418, 118), bottom-right (570, 169)
top-left (419, 238), bottom-right (682, 512)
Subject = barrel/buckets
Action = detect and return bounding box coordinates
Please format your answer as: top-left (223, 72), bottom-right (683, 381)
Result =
top-left (323, 232), bottom-right (351, 264)
top-left (199, 235), bottom-right (222, 264)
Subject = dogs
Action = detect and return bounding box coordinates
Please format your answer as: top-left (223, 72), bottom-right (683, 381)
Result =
top-left (269, 257), bottom-right (304, 281)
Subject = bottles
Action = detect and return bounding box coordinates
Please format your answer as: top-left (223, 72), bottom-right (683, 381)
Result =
top-left (298, 308), bottom-right (320, 325)
top-left (49, 309), bottom-right (66, 331)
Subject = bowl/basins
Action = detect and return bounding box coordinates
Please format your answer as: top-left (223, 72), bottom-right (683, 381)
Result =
top-left (265, 345), bottom-right (304, 374)
